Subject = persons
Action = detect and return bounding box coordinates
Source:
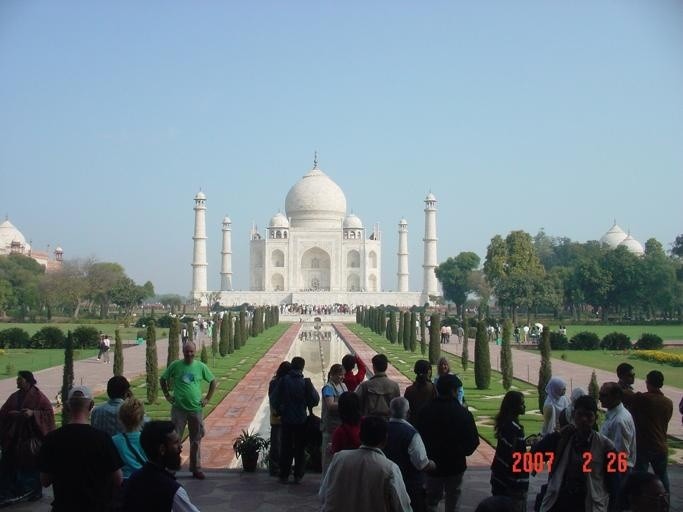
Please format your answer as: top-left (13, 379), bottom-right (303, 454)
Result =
top-left (486, 322), bottom-right (569, 345)
top-left (97, 329), bottom-right (112, 365)
top-left (416, 316), bottom-right (466, 344)
top-left (278, 302), bottom-right (367, 315)
top-left (268, 355), bottom-right (479, 512)
top-left (0, 369), bottom-right (202, 512)
top-left (159, 341), bottom-right (217, 480)
top-left (473, 362), bottom-right (683, 512)
top-left (170, 299), bottom-right (272, 344)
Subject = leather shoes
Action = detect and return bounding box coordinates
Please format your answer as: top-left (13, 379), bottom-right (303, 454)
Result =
top-left (194, 472), bottom-right (204, 478)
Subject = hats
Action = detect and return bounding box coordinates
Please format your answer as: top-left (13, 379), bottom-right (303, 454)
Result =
top-left (66, 385), bottom-right (92, 400)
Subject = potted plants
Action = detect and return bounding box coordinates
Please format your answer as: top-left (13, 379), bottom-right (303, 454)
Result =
top-left (232, 427), bottom-right (268, 472)
